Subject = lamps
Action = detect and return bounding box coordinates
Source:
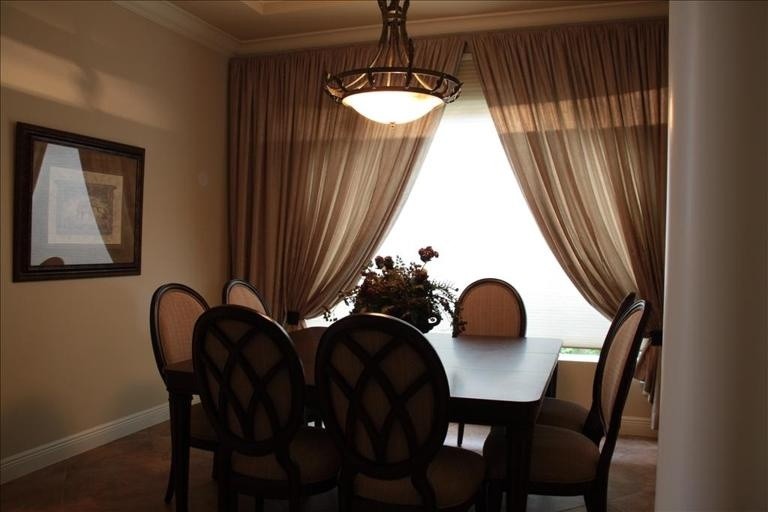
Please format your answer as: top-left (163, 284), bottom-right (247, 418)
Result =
top-left (322, 1), bottom-right (463, 129)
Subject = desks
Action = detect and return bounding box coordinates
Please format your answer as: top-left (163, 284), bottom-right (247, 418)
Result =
top-left (161, 326), bottom-right (563, 510)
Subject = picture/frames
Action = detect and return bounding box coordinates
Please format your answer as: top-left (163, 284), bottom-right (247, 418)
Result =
top-left (14, 120), bottom-right (144, 283)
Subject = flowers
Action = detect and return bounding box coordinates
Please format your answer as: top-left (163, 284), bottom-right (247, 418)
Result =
top-left (321, 245), bottom-right (468, 338)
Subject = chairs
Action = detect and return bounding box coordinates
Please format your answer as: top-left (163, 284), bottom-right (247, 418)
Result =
top-left (481, 300), bottom-right (652, 510)
top-left (222, 278), bottom-right (272, 317)
top-left (152, 277), bottom-right (265, 510)
top-left (190, 304), bottom-right (339, 510)
top-left (490, 291), bottom-right (636, 443)
top-left (455, 282), bottom-right (527, 448)
top-left (312, 312), bottom-right (489, 510)
